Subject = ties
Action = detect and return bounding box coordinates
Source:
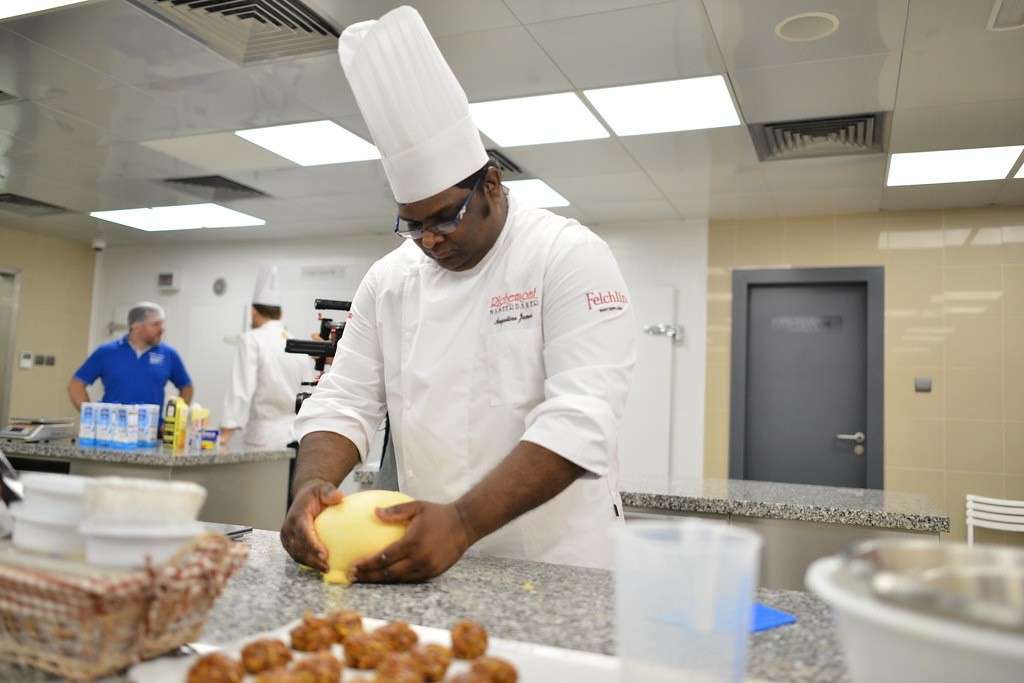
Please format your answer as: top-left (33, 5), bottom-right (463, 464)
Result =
top-left (253, 277), bottom-right (284, 307)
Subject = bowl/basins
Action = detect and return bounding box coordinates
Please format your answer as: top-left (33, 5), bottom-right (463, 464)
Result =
top-left (807, 541), bottom-right (1024, 683)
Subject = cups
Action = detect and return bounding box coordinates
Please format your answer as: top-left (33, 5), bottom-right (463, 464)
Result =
top-left (613, 520), bottom-right (760, 683)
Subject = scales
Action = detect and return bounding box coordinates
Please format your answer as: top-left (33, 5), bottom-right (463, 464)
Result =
top-left (0, 415), bottom-right (77, 443)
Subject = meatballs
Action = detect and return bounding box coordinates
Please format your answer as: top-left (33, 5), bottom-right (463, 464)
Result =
top-left (188, 611), bottom-right (518, 683)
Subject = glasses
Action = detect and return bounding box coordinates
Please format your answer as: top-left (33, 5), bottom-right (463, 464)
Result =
top-left (395, 177), bottom-right (483, 240)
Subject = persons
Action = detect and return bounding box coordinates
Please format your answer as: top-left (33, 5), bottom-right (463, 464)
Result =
top-left (278, 122), bottom-right (630, 584)
top-left (215, 292), bottom-right (299, 513)
top-left (65, 301), bottom-right (196, 438)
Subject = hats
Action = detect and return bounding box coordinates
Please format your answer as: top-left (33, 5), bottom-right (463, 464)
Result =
top-left (339, 6), bottom-right (487, 202)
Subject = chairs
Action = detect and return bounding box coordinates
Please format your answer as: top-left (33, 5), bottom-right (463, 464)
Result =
top-left (965, 494), bottom-right (1024, 549)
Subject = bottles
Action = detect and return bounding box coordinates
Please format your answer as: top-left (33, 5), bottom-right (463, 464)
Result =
top-left (185, 402), bottom-right (201, 457)
